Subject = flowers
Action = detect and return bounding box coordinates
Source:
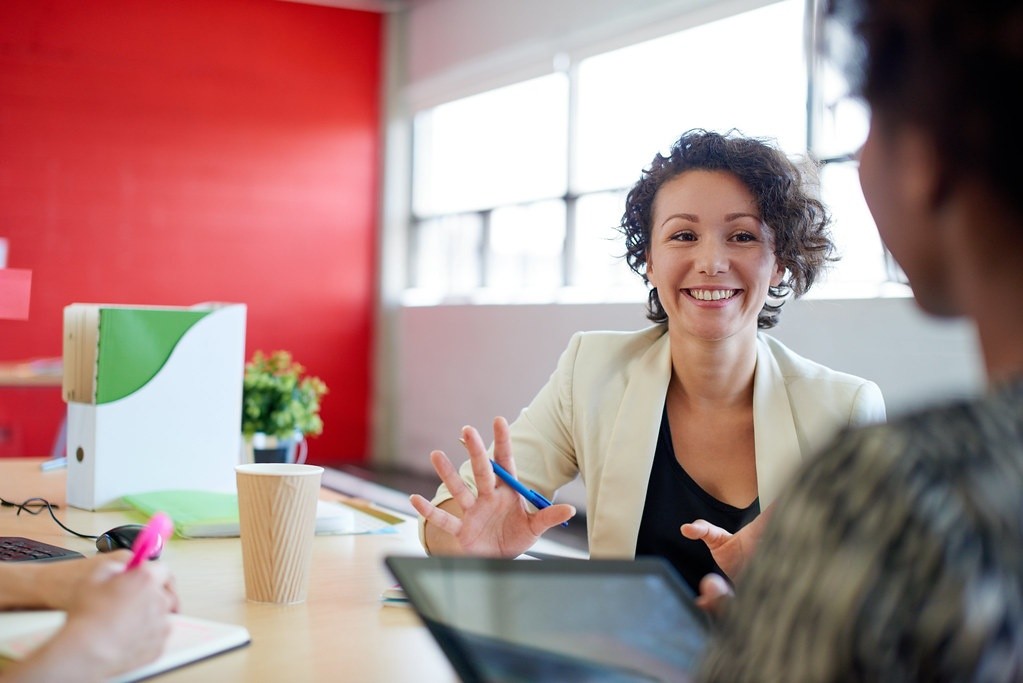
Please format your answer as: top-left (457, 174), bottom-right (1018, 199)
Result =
top-left (239, 350), bottom-right (330, 439)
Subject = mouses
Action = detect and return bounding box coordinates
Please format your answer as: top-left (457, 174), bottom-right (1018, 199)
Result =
top-left (94, 521), bottom-right (160, 559)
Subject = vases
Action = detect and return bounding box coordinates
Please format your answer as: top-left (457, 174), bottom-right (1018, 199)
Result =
top-left (241, 432), bottom-right (302, 464)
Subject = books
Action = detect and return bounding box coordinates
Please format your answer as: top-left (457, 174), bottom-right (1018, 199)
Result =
top-left (0, 610), bottom-right (251, 683)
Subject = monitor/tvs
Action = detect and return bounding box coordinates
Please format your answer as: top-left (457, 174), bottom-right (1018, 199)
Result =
top-left (388, 548), bottom-right (718, 683)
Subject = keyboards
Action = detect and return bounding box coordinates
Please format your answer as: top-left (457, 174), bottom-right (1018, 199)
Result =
top-left (0, 535), bottom-right (87, 563)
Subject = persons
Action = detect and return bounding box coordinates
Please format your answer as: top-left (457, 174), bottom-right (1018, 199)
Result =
top-left (0, 549), bottom-right (177, 683)
top-left (408, 129), bottom-right (885, 593)
top-left (696, 0), bottom-right (1023, 683)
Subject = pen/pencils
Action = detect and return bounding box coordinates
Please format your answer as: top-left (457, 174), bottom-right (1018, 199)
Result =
top-left (38, 457), bottom-right (67, 473)
top-left (125, 511), bottom-right (176, 573)
top-left (458, 436), bottom-right (568, 529)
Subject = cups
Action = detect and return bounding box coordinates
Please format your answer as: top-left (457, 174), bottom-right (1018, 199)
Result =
top-left (236, 463), bottom-right (325, 604)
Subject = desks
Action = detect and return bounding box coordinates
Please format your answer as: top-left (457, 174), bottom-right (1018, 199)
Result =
top-left (0, 454), bottom-right (537, 683)
